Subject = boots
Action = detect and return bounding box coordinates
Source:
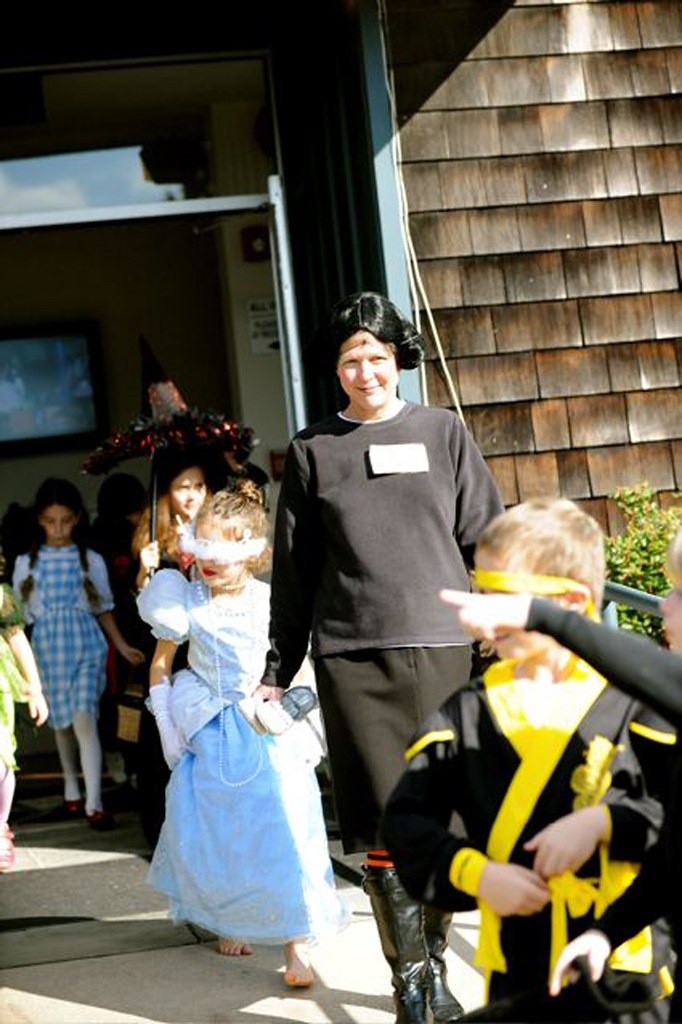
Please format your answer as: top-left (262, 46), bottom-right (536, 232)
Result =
top-left (361, 869), bottom-right (428, 1024)
top-left (424, 906), bottom-right (462, 1024)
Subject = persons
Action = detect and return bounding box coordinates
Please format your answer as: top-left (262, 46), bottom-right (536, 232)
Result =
top-left (439, 514), bottom-right (682, 1024)
top-left (382, 494), bottom-right (679, 1024)
top-left (0, 421), bottom-right (271, 871)
top-left (135, 477), bottom-right (341, 987)
top-left (241, 289), bottom-right (504, 1024)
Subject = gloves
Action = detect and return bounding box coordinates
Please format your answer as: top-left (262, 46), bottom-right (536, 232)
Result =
top-left (150, 685), bottom-right (183, 769)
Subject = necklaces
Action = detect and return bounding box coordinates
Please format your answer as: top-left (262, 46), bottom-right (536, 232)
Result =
top-left (195, 576), bottom-right (264, 787)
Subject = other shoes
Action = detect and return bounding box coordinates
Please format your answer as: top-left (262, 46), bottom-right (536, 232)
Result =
top-left (89, 811), bottom-right (111, 828)
top-left (238, 697), bottom-right (266, 734)
top-left (0, 822), bottom-right (13, 868)
top-left (64, 796), bottom-right (84, 813)
top-left (257, 686), bottom-right (317, 735)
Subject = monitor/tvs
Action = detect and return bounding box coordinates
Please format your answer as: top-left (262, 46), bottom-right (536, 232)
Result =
top-left (0, 315), bottom-right (111, 461)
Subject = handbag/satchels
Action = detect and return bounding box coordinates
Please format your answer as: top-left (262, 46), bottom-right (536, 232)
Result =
top-left (117, 658), bottom-right (150, 743)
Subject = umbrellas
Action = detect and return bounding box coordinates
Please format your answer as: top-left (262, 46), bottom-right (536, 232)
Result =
top-left (73, 382), bottom-right (260, 580)
